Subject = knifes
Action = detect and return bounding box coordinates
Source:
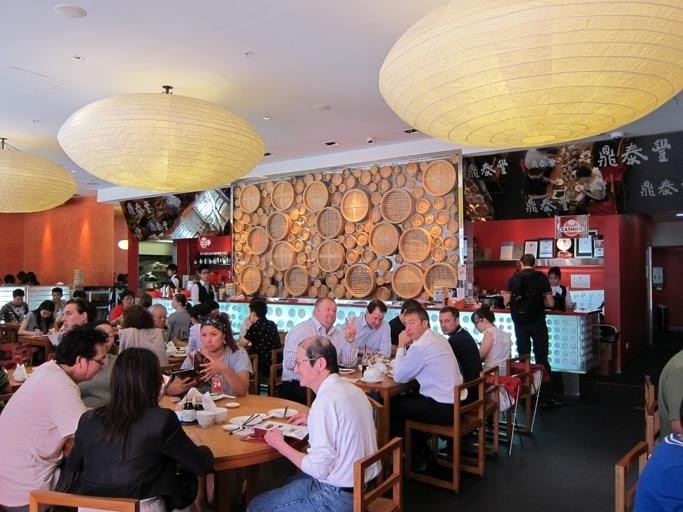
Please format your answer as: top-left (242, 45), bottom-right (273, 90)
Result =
top-left (283, 407), bottom-right (287, 419)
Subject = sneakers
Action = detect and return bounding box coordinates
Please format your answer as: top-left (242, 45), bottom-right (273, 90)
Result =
top-left (427, 438), bottom-right (448, 456)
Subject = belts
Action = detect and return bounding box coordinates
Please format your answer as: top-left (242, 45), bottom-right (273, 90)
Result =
top-left (340, 487), bottom-right (353, 493)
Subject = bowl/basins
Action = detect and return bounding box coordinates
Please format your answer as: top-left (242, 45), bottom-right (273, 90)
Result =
top-left (196, 409), bottom-right (227, 428)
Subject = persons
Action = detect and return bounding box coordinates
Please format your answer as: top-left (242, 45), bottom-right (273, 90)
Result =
top-left (245, 337), bottom-right (383, 512)
top-left (504, 254), bottom-right (563, 411)
top-left (164, 310), bottom-right (254, 512)
top-left (112, 274), bottom-right (128, 304)
top-left (282, 297), bottom-right (360, 406)
top-left (544, 266), bottom-right (575, 312)
top-left (657, 350), bottom-right (683, 441)
top-left (191, 264), bottom-right (218, 307)
top-left (1, 324), bottom-right (108, 512)
top-left (239, 297), bottom-right (281, 377)
top-left (631, 398), bottom-right (683, 512)
top-left (2, 286), bottom-right (220, 409)
top-left (166, 264), bottom-right (181, 290)
top-left (4, 271), bottom-right (40, 286)
top-left (352, 298), bottom-right (511, 473)
top-left (66, 347), bottom-right (214, 511)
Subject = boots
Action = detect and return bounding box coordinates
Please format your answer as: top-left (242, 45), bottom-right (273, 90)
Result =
top-left (540, 380), bottom-right (563, 408)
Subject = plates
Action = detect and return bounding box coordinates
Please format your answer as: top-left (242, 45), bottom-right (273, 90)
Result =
top-left (268, 409), bottom-right (297, 418)
top-left (210, 392), bottom-right (223, 401)
top-left (338, 369), bottom-right (354, 375)
top-left (228, 415), bottom-right (262, 426)
top-left (222, 424), bottom-right (238, 432)
top-left (362, 378), bottom-right (383, 383)
top-left (225, 403), bottom-right (240, 407)
top-left (169, 354), bottom-right (187, 359)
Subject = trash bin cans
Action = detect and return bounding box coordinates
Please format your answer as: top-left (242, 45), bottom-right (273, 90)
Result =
top-left (657, 305), bottom-right (668, 334)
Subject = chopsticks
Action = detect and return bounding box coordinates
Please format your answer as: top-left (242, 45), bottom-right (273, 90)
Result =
top-left (242, 414), bottom-right (259, 425)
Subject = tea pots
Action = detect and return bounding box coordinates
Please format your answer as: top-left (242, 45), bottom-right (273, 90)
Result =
top-left (363, 357), bottom-right (383, 381)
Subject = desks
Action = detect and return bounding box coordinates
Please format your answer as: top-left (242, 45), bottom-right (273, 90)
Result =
top-left (1, 366), bottom-right (40, 393)
top-left (159, 394), bottom-right (311, 511)
top-left (306, 354), bottom-right (412, 444)
top-left (17, 335), bottom-right (52, 362)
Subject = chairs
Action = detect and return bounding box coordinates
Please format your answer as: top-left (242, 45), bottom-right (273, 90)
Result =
top-left (615, 441), bottom-right (650, 512)
top-left (649, 400), bottom-right (660, 457)
top-left (0, 323), bottom-right (532, 512)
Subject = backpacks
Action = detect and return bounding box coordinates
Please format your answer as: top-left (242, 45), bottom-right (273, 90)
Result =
top-left (511, 271), bottom-right (541, 323)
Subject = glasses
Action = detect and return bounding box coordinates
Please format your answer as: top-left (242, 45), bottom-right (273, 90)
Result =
top-left (296, 358), bottom-right (316, 367)
top-left (94, 359), bottom-right (106, 368)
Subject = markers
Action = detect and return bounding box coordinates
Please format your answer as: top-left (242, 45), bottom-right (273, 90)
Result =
top-left (229, 426), bottom-right (246, 435)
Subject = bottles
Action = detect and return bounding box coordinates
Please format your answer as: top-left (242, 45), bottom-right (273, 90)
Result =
top-left (183, 397), bottom-right (194, 424)
top-left (362, 353), bottom-right (368, 373)
top-left (472, 237), bottom-right (477, 260)
top-left (161, 284), bottom-right (169, 298)
top-left (194, 256), bottom-right (229, 265)
top-left (195, 398), bottom-right (203, 421)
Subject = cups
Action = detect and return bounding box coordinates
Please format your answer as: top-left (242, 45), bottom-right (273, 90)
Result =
top-left (178, 328), bottom-right (186, 341)
top-left (218, 289), bottom-right (225, 301)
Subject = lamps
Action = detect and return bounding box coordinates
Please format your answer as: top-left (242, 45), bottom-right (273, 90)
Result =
top-left (57, 86), bottom-right (266, 194)
top-left (0, 138), bottom-right (77, 214)
top-left (378, 0), bottom-right (683, 149)
